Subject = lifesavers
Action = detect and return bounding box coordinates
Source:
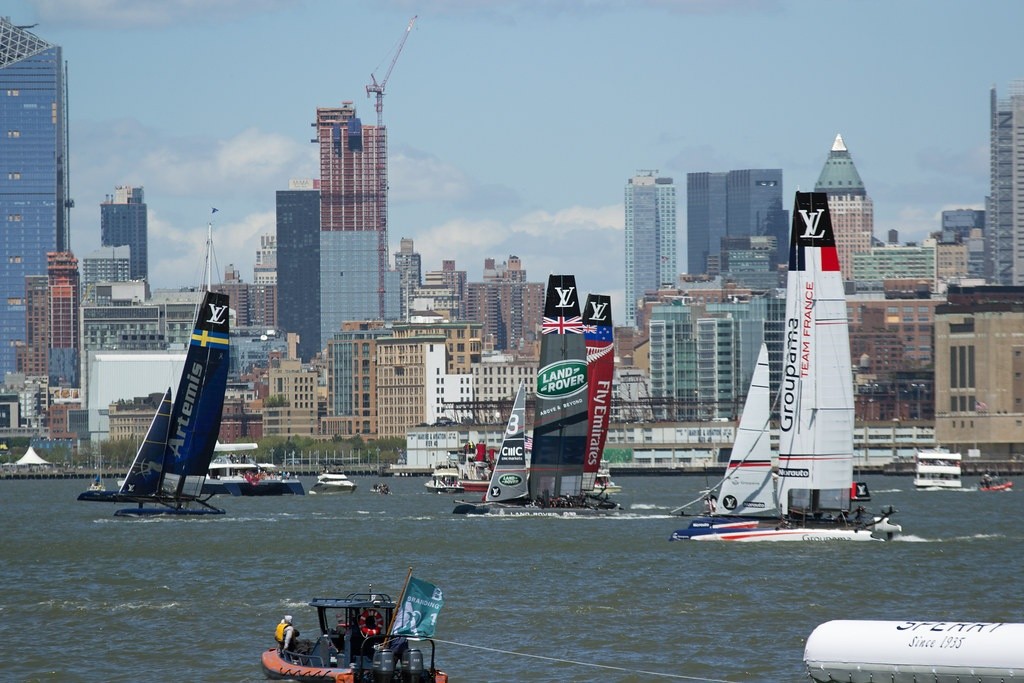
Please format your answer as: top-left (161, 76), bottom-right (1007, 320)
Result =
top-left (359, 608), bottom-right (383, 634)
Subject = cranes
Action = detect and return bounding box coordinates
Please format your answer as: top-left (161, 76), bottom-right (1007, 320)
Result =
top-left (364, 13), bottom-right (417, 316)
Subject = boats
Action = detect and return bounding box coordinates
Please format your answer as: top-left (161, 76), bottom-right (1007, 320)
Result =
top-left (78, 440), bottom-right (306, 501)
top-left (978, 481), bottom-right (1013, 491)
top-left (914, 451), bottom-right (963, 488)
top-left (451, 441), bottom-right (497, 492)
top-left (262, 585), bottom-right (447, 683)
top-left (309, 469), bottom-right (357, 495)
top-left (369, 481), bottom-right (392, 495)
top-left (424, 463), bottom-right (465, 493)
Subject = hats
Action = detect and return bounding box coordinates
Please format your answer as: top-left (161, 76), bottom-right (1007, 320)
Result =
top-left (336, 614), bottom-right (343, 622)
top-left (284, 614), bottom-right (292, 624)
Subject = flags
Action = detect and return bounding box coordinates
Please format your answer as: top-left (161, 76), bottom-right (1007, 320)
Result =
top-left (975, 400), bottom-right (989, 413)
top-left (390, 575), bottom-right (445, 641)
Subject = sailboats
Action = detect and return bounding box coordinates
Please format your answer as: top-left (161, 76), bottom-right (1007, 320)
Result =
top-left (453, 275), bottom-right (623, 516)
top-left (112, 222), bottom-right (231, 518)
top-left (670, 186), bottom-right (901, 542)
top-left (88, 422), bottom-right (106, 491)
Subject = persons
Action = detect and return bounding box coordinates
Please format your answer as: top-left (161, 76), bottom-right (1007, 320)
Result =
top-left (230, 452), bottom-right (247, 464)
top-left (262, 469), bottom-right (290, 480)
top-left (275, 614), bottom-right (296, 657)
top-left (373, 479), bottom-right (390, 495)
top-left (981, 474), bottom-right (998, 488)
